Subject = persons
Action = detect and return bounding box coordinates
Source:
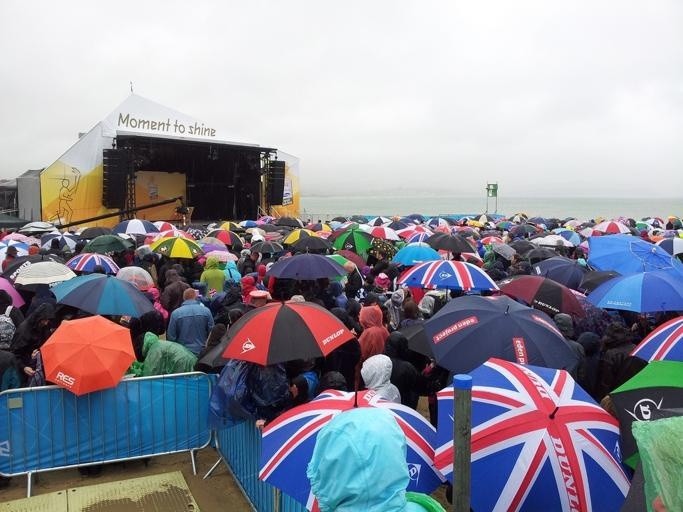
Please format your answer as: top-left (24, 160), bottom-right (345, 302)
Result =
top-left (175, 195), bottom-right (188, 214)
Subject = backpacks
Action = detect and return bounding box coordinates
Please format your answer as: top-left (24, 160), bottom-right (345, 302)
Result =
top-left (0, 303), bottom-right (14, 348)
top-left (422, 294), bottom-right (441, 318)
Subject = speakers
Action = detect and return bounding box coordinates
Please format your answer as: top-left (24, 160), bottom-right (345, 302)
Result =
top-left (268, 160), bottom-right (286, 205)
top-left (102, 148), bottom-right (127, 209)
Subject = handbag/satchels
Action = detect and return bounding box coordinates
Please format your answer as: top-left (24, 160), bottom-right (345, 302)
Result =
top-left (27, 351), bottom-right (45, 387)
